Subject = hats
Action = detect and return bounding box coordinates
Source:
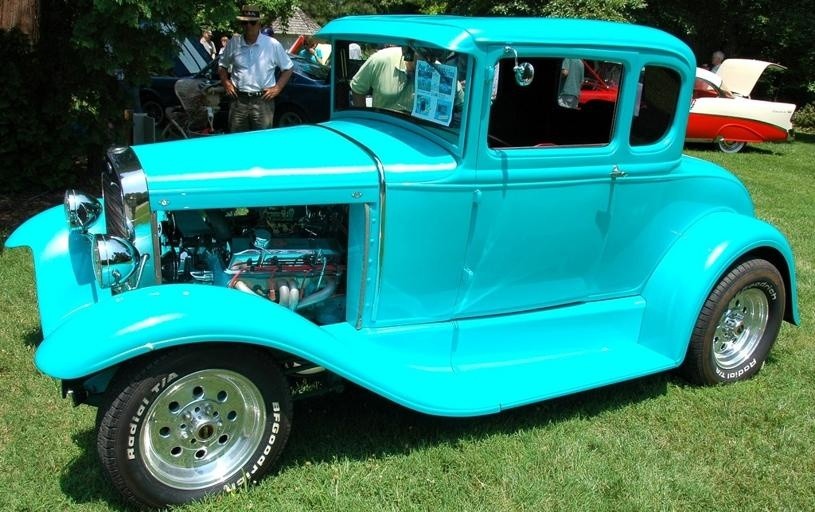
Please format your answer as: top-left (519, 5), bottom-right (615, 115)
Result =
top-left (236, 4), bottom-right (261, 21)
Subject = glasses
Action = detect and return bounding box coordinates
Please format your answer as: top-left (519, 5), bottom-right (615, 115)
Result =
top-left (242, 21), bottom-right (257, 26)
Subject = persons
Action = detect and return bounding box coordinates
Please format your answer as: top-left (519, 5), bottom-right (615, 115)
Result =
top-left (556, 57), bottom-right (586, 110)
top-left (702, 50), bottom-right (725, 73)
top-left (216, 3), bottom-right (295, 132)
top-left (349, 44), bottom-right (464, 116)
top-left (219, 35), bottom-right (234, 74)
top-left (200, 27), bottom-right (217, 63)
top-left (262, 26), bottom-right (274, 37)
top-left (299, 35), bottom-right (325, 67)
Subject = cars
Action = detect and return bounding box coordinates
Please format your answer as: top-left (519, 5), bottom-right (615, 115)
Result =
top-left (578, 59), bottom-right (798, 154)
top-left (138, 21), bottom-right (355, 129)
top-left (5, 15), bottom-right (801, 512)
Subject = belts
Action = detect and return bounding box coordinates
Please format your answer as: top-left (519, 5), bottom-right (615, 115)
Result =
top-left (248, 92), bottom-right (262, 96)
